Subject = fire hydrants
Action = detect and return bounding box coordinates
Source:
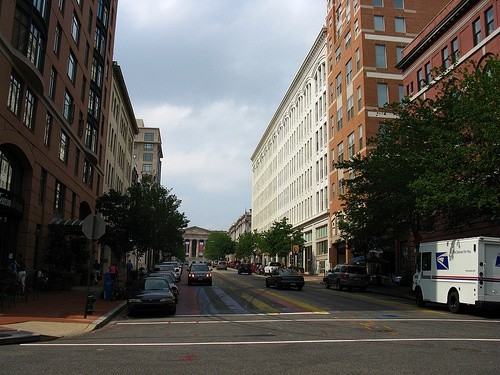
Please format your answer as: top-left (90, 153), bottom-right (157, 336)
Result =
top-left (86, 289), bottom-right (98, 316)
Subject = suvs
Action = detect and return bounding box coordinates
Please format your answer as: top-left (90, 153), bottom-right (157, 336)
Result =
top-left (186, 263), bottom-right (213, 286)
top-left (324, 263), bottom-right (369, 292)
top-left (210, 259), bottom-right (282, 276)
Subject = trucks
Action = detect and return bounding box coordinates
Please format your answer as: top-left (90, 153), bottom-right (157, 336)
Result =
top-left (411, 236), bottom-right (500, 315)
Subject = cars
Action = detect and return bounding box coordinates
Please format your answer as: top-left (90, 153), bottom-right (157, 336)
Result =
top-left (126, 276), bottom-right (177, 317)
top-left (143, 259), bottom-right (185, 297)
top-left (266, 267), bottom-right (305, 291)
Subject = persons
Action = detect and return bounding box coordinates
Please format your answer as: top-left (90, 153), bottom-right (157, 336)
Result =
top-left (16, 253), bottom-right (26, 292)
top-left (110, 263), bottom-right (116, 273)
top-left (127, 260), bottom-right (133, 280)
top-left (93, 260), bottom-right (100, 285)
